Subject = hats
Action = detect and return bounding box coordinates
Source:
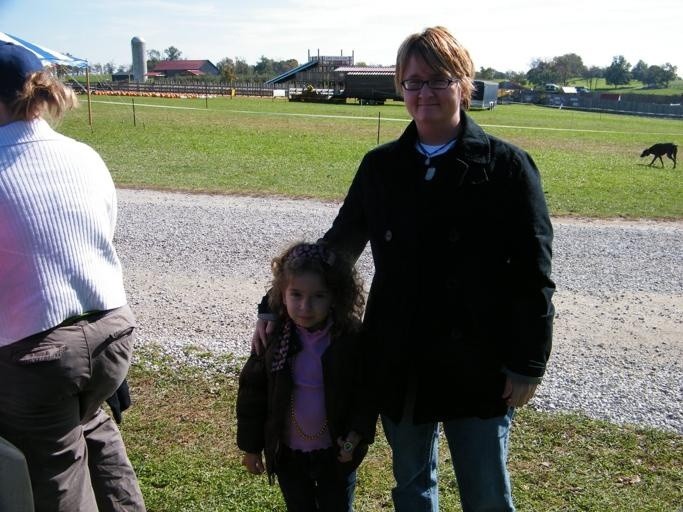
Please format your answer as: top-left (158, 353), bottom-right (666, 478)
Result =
top-left (0, 41), bottom-right (44, 103)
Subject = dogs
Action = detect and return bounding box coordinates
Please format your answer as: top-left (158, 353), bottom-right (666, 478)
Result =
top-left (640, 143), bottom-right (678, 169)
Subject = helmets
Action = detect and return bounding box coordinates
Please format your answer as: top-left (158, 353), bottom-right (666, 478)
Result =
top-left (400, 78), bottom-right (459, 91)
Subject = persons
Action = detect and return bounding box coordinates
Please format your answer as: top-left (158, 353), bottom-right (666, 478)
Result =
top-left (0, 42), bottom-right (146, 512)
